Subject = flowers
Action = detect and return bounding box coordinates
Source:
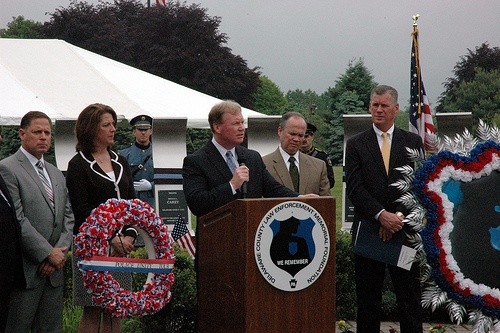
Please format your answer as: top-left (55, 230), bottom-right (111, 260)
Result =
top-left (74, 198), bottom-right (176, 318)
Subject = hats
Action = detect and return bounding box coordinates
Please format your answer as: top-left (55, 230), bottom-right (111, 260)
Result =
top-left (130, 115), bottom-right (152, 130)
top-left (304, 123), bottom-right (318, 136)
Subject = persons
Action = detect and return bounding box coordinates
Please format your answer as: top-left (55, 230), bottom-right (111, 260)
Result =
top-left (345, 85), bottom-right (425, 333)
top-left (299, 123), bottom-right (335, 189)
top-left (182, 100), bottom-right (319, 308)
top-left (0, 111), bottom-right (75, 333)
top-left (117, 115), bottom-right (155, 207)
top-left (66, 103), bottom-right (138, 333)
top-left (0, 135), bottom-right (27, 333)
top-left (262, 112), bottom-right (332, 196)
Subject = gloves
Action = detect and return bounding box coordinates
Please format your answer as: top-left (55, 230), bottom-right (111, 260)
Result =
top-left (133, 179), bottom-right (152, 191)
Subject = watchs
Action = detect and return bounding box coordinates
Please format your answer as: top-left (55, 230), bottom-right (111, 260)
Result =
top-left (395, 211), bottom-right (406, 218)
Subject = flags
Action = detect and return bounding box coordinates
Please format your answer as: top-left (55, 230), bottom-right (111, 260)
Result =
top-left (409, 14), bottom-right (440, 152)
top-left (172, 215), bottom-right (195, 259)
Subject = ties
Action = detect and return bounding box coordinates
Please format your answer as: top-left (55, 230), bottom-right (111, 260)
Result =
top-left (288, 157), bottom-right (299, 193)
top-left (226, 151), bottom-right (238, 175)
top-left (381, 132), bottom-right (390, 176)
top-left (35, 161), bottom-right (54, 209)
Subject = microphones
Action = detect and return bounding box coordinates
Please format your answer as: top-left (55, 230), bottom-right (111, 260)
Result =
top-left (237, 155), bottom-right (248, 198)
